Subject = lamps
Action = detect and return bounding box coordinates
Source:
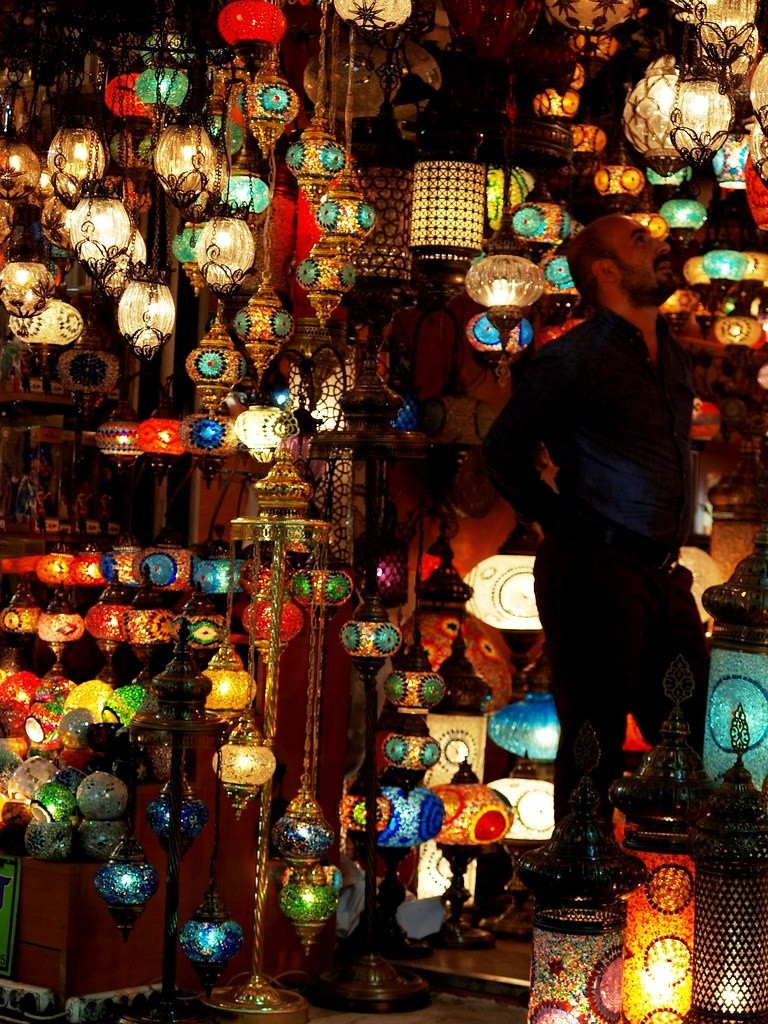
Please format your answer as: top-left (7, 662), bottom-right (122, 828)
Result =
top-left (0, 0), bottom-right (768, 1024)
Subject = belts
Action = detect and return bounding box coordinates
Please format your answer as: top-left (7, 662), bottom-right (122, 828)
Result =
top-left (556, 510), bottom-right (674, 572)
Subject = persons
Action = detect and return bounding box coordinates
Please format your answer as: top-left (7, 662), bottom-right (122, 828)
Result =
top-left (480, 216), bottom-right (712, 828)
